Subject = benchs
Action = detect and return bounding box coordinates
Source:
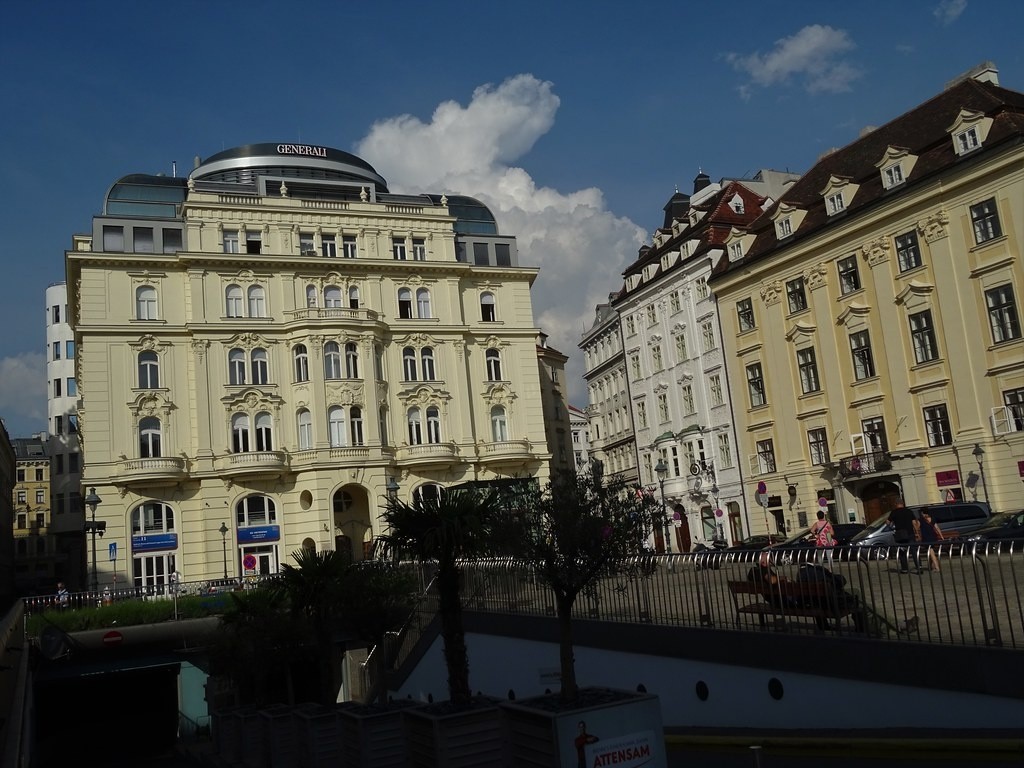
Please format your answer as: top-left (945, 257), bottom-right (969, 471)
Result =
top-left (727, 579), bottom-right (863, 638)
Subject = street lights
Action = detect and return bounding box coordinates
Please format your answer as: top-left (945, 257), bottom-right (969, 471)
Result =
top-left (655, 458), bottom-right (675, 574)
top-left (218, 522), bottom-right (229, 579)
top-left (972, 443), bottom-right (991, 511)
top-left (711, 484), bottom-right (726, 542)
top-left (86, 487), bottom-right (102, 593)
top-left (387, 477), bottom-right (401, 561)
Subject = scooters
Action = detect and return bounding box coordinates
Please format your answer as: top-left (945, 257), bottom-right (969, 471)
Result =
top-left (690, 536), bottom-right (728, 570)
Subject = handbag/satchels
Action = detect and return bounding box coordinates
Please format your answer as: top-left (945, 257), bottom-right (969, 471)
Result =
top-left (812, 533), bottom-right (819, 540)
top-left (935, 532), bottom-right (942, 541)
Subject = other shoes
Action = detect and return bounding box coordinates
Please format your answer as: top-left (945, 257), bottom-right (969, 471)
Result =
top-left (913, 565), bottom-right (921, 569)
top-left (899, 569), bottom-right (907, 574)
top-left (933, 568), bottom-right (940, 572)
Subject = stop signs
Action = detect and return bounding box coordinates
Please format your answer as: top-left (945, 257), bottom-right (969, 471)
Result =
top-left (102, 631), bottom-right (124, 647)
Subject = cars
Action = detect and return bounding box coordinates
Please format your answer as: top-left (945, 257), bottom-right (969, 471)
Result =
top-left (961, 510), bottom-right (1024, 553)
top-left (725, 534), bottom-right (788, 562)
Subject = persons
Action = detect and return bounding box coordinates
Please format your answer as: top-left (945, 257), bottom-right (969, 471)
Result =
top-left (795, 548), bottom-right (920, 636)
top-left (811, 511), bottom-right (835, 572)
top-left (55, 582), bottom-right (70, 610)
top-left (914, 506), bottom-right (944, 572)
top-left (748, 551), bottom-right (832, 630)
top-left (575, 721), bottom-right (599, 768)
top-left (886, 498), bottom-right (924, 574)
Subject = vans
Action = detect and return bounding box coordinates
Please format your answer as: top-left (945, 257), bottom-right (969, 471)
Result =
top-left (760, 523), bottom-right (867, 566)
top-left (848, 501), bottom-right (990, 560)
